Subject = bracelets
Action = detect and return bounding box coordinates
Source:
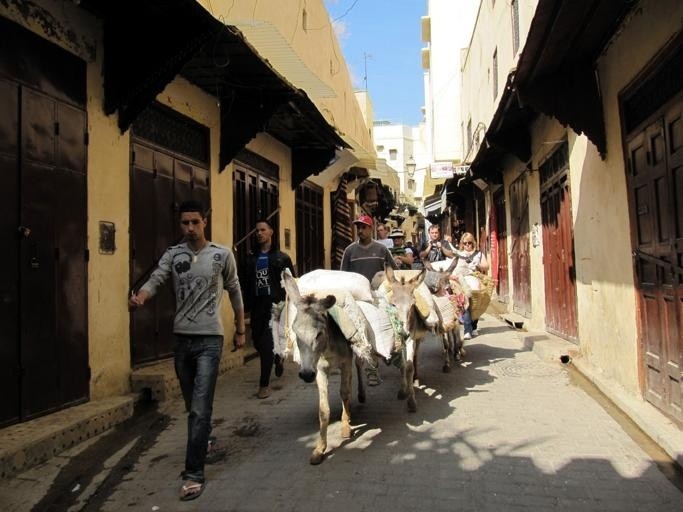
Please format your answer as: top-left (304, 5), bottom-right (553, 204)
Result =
top-left (235, 330), bottom-right (245, 336)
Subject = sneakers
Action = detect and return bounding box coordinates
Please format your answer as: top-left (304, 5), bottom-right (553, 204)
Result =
top-left (274, 356), bottom-right (285, 376)
top-left (364, 367), bottom-right (384, 386)
top-left (257, 386), bottom-right (270, 399)
top-left (463, 329), bottom-right (479, 340)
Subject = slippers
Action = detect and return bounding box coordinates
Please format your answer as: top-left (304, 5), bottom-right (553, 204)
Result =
top-left (180, 478), bottom-right (208, 500)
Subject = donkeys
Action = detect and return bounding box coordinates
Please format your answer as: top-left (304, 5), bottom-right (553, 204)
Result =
top-left (277, 270), bottom-right (367, 466)
top-left (383, 265), bottom-right (432, 416)
top-left (421, 255), bottom-right (466, 373)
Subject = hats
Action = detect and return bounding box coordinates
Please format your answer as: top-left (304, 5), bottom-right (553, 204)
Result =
top-left (387, 228), bottom-right (406, 239)
top-left (352, 214), bottom-right (375, 228)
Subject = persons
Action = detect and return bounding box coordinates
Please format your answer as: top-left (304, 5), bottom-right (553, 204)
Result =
top-left (374, 221), bottom-right (453, 270)
top-left (452, 230), bottom-right (489, 341)
top-left (123, 198), bottom-right (247, 499)
top-left (338, 215), bottom-right (400, 386)
top-left (240, 216), bottom-right (303, 399)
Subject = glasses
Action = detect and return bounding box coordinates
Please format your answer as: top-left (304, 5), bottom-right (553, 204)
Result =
top-left (462, 241), bottom-right (473, 245)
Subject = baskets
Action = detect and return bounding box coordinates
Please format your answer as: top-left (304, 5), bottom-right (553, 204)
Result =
top-left (470, 271), bottom-right (499, 323)
